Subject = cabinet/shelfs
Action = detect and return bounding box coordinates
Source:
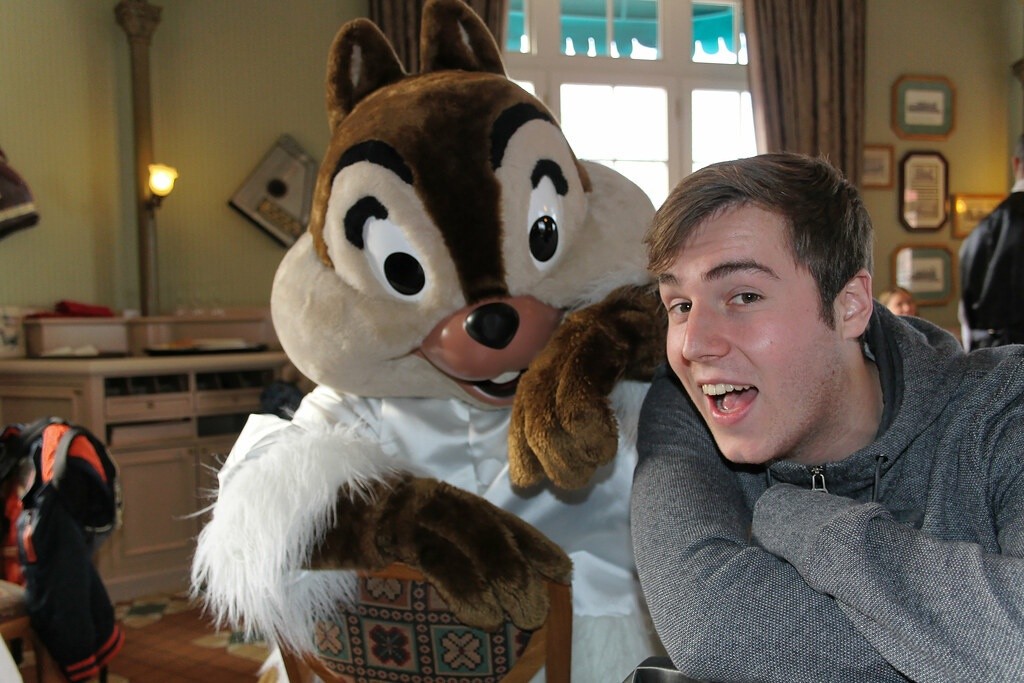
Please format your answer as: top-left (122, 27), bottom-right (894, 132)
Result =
top-left (0, 367), bottom-right (288, 606)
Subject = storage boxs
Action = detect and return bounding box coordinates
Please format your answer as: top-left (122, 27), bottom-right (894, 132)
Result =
top-left (24, 317), bottom-right (128, 357)
top-left (127, 316), bottom-right (263, 353)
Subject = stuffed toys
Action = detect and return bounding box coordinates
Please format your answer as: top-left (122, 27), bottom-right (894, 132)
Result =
top-left (191, 0), bottom-right (679, 683)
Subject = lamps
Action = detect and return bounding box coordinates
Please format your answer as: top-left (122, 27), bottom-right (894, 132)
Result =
top-left (144, 164), bottom-right (178, 211)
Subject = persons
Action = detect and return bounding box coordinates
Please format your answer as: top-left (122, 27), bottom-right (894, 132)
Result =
top-left (879, 287), bottom-right (916, 318)
top-left (957, 133), bottom-right (1024, 350)
top-left (630, 153), bottom-right (1024, 682)
top-left (0, 413), bottom-right (126, 682)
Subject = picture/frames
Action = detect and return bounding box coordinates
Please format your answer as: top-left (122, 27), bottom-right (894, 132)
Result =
top-left (892, 75), bottom-right (956, 139)
top-left (892, 243), bottom-right (957, 305)
top-left (898, 150), bottom-right (950, 232)
top-left (861, 143), bottom-right (894, 189)
top-left (952, 193), bottom-right (1007, 239)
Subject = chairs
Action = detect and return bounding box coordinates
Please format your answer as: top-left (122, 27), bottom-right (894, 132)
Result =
top-left (0, 423), bottom-right (117, 683)
top-left (273, 561), bottom-right (573, 683)
